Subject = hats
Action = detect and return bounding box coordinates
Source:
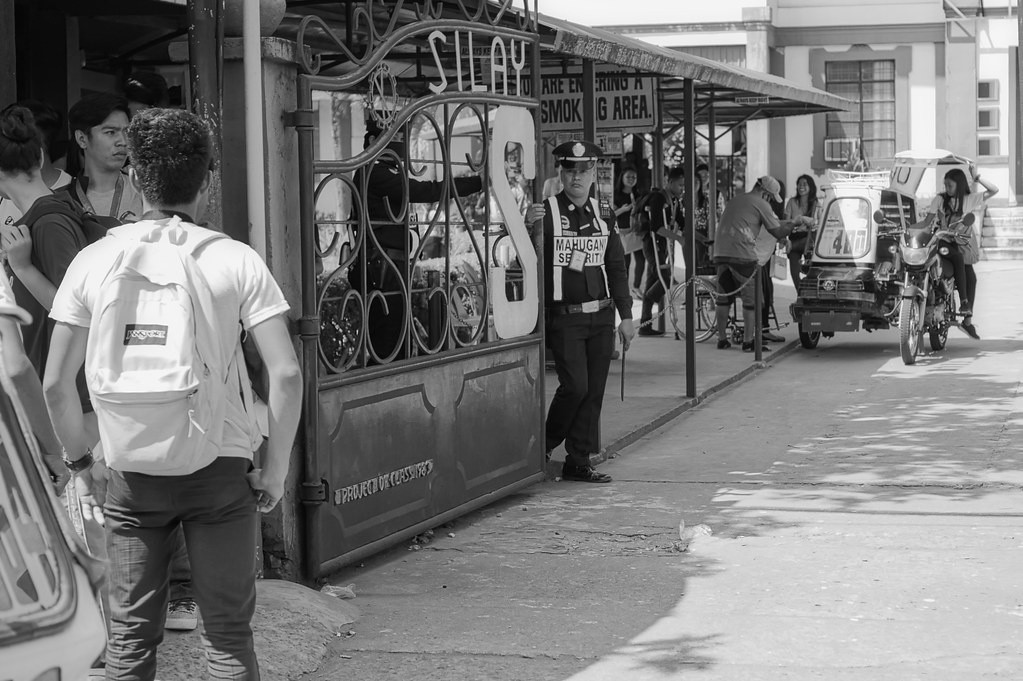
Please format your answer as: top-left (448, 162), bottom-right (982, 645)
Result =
top-left (121, 71), bottom-right (167, 106)
top-left (366, 107), bottom-right (411, 132)
top-left (551, 140), bottom-right (603, 171)
top-left (757, 176), bottom-right (783, 203)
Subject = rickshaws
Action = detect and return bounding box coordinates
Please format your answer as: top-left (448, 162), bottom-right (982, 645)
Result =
top-left (789, 148), bottom-right (977, 365)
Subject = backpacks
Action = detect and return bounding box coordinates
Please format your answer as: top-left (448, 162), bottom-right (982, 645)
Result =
top-left (630, 189), bottom-right (678, 234)
top-left (84, 217), bottom-right (235, 476)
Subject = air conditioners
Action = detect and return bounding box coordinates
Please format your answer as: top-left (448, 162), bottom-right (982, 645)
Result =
top-left (824, 137), bottom-right (860, 162)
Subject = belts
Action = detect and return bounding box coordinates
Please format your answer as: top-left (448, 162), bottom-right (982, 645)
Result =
top-left (547, 299), bottom-right (613, 312)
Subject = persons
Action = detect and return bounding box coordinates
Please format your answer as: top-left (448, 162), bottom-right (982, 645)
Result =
top-left (696, 165), bottom-right (724, 222)
top-left (612, 166), bottom-right (645, 300)
top-left (524, 141), bottom-right (634, 483)
top-left (351, 109), bottom-right (486, 365)
top-left (909, 166), bottom-right (999, 340)
top-left (464, 192), bottom-right (486, 225)
top-left (712, 175), bottom-right (822, 351)
top-left (0, 92), bottom-right (200, 631)
top-left (43, 107), bottom-right (304, 681)
top-left (637, 168), bottom-right (686, 338)
top-left (670, 174), bottom-right (709, 246)
top-left (543, 161), bottom-right (564, 201)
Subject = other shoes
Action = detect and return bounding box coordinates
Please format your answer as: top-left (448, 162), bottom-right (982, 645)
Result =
top-left (762, 331), bottom-right (785, 342)
top-left (716, 339), bottom-right (732, 349)
top-left (741, 340), bottom-right (755, 351)
top-left (631, 288), bottom-right (644, 300)
top-left (638, 327), bottom-right (664, 336)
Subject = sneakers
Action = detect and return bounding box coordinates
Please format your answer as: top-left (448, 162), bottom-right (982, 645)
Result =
top-left (959, 321), bottom-right (980, 341)
top-left (959, 302), bottom-right (972, 315)
top-left (164, 599), bottom-right (199, 629)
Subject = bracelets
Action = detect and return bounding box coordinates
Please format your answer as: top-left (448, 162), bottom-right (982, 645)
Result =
top-left (63, 448), bottom-right (93, 471)
top-left (974, 174), bottom-right (980, 182)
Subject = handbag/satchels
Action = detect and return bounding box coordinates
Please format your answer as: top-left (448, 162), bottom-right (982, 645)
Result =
top-left (768, 248), bottom-right (787, 280)
top-left (618, 228), bottom-right (645, 255)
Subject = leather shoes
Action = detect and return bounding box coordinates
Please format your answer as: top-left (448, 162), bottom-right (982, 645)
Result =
top-left (562, 461), bottom-right (612, 482)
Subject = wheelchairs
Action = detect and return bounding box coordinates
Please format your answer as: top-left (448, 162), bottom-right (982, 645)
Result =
top-left (668, 240), bottom-right (789, 344)
